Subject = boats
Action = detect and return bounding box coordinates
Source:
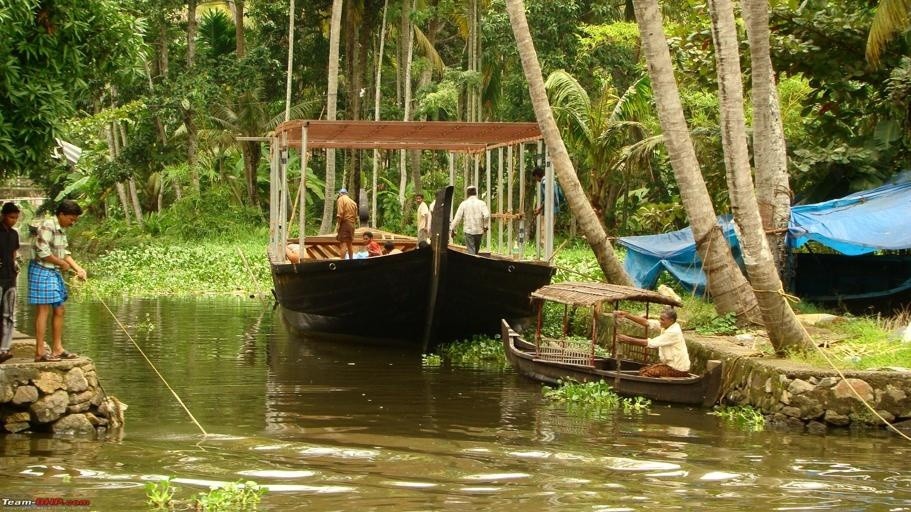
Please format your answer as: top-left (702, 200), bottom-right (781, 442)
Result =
top-left (256, 114), bottom-right (562, 341)
top-left (793, 251), bottom-right (911, 309)
top-left (498, 280), bottom-right (725, 411)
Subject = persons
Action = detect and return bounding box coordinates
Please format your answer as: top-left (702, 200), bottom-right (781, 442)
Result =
top-left (533, 167), bottom-right (560, 249)
top-left (27, 201), bottom-right (87, 362)
top-left (449, 187), bottom-right (489, 255)
top-left (429, 191), bottom-right (440, 242)
top-left (415, 193), bottom-right (429, 248)
top-left (0, 202), bottom-right (20, 363)
top-left (363, 231), bottom-right (383, 255)
top-left (384, 240), bottom-right (403, 255)
top-left (613, 307), bottom-right (691, 377)
top-left (335, 188), bottom-right (358, 259)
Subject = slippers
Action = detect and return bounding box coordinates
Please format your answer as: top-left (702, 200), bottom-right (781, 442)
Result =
top-left (34, 353), bottom-right (62, 362)
top-left (55, 350), bottom-right (79, 359)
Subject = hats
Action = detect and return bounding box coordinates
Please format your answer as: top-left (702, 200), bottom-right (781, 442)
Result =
top-left (339, 188), bottom-right (347, 193)
